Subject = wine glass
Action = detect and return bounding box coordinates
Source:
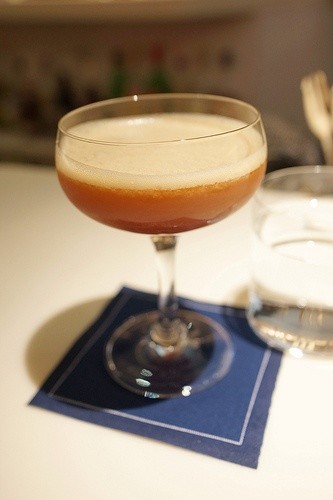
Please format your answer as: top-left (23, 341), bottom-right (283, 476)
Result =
top-left (55, 93), bottom-right (269, 400)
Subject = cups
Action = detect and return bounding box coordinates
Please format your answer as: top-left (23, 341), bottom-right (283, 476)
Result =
top-left (237, 166), bottom-right (332, 360)
